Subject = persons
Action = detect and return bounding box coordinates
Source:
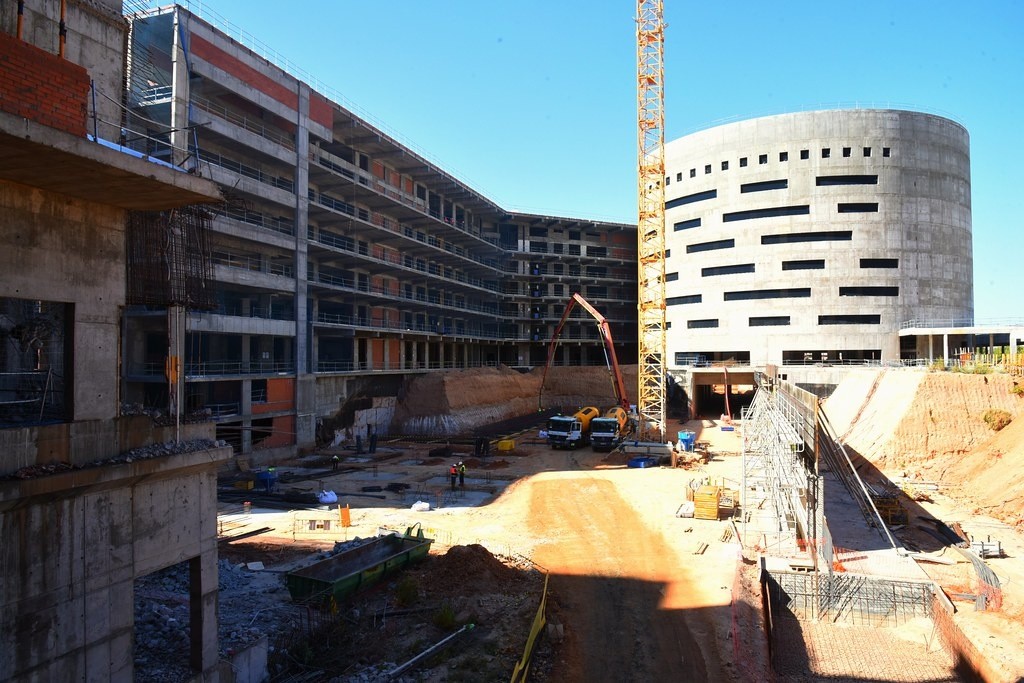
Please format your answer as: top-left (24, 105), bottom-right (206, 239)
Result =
top-left (451, 461), bottom-right (465, 491)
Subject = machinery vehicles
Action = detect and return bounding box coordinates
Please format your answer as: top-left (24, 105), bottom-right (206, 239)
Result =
top-left (539, 293), bottom-right (642, 421)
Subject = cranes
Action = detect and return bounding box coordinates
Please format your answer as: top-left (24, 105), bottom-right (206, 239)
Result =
top-left (624, 0), bottom-right (675, 456)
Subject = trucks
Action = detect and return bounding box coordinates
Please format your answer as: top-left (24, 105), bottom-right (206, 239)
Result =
top-left (589, 405), bottom-right (635, 451)
top-left (545, 407), bottom-right (600, 450)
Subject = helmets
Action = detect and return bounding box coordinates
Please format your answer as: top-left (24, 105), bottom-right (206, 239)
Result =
top-left (452, 464), bottom-right (456, 466)
top-left (459, 462), bottom-right (462, 465)
